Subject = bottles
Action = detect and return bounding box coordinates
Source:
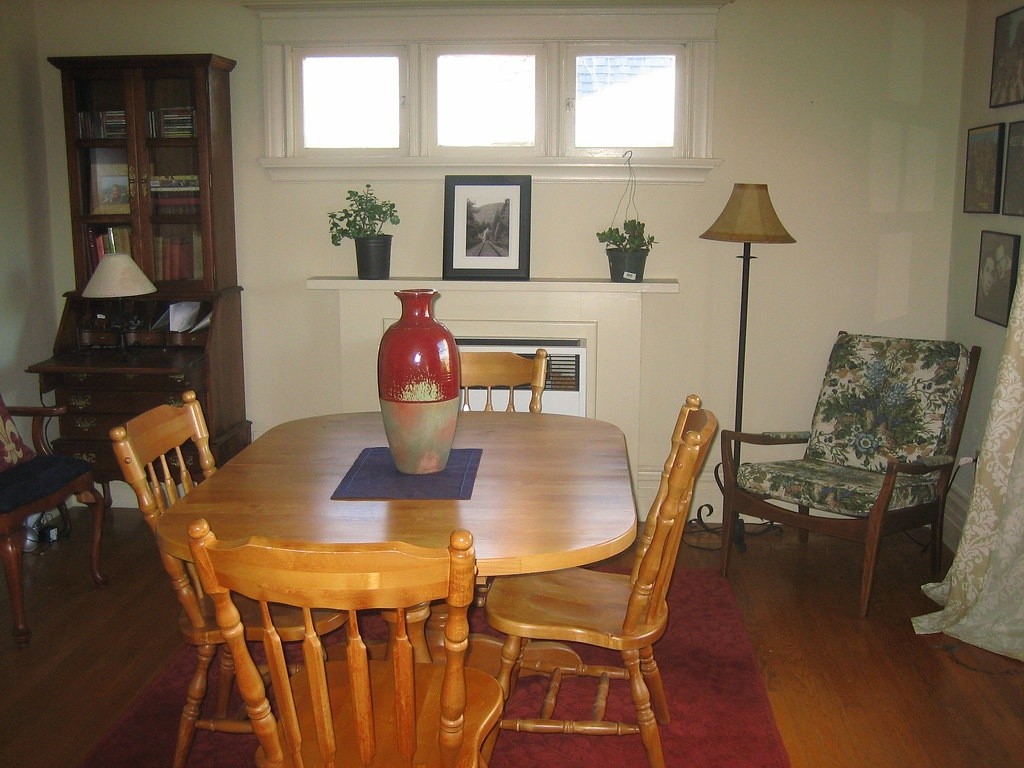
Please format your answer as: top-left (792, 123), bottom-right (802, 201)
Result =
top-left (378, 288), bottom-right (461, 474)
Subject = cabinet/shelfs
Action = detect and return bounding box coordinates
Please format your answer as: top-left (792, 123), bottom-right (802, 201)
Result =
top-left (23, 52), bottom-right (252, 537)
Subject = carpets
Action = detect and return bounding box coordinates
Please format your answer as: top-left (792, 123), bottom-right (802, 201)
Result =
top-left (78, 570), bottom-right (796, 768)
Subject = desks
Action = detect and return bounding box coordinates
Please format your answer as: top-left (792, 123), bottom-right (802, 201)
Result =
top-left (154, 409), bottom-right (640, 667)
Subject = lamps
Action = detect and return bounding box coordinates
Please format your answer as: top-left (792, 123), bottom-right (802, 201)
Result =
top-left (81, 254), bottom-right (158, 345)
top-left (700, 182), bottom-right (797, 555)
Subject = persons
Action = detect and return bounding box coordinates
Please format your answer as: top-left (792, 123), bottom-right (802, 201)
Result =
top-left (102, 184), bottom-right (127, 203)
top-left (976, 241), bottom-right (1012, 325)
top-left (482, 235), bottom-right (486, 245)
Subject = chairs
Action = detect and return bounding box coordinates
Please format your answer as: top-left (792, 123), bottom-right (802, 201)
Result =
top-left (719, 329), bottom-right (984, 620)
top-left (486, 394), bottom-right (719, 768)
top-left (455, 348), bottom-right (551, 412)
top-left (109, 389), bottom-right (352, 768)
top-left (180, 517), bottom-right (508, 768)
top-left (1, 391), bottom-right (115, 647)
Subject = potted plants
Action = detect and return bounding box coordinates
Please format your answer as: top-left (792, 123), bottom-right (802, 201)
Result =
top-left (326, 184), bottom-right (403, 280)
top-left (595, 220), bottom-right (658, 283)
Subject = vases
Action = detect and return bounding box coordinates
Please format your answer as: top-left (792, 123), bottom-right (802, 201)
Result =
top-left (377, 287), bottom-right (462, 472)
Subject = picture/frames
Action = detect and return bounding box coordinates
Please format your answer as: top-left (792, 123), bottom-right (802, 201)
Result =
top-left (975, 225), bottom-right (1021, 328)
top-left (441, 173), bottom-right (534, 283)
top-left (88, 165), bottom-right (155, 215)
top-left (989, 6), bottom-right (1024, 109)
top-left (1001, 119), bottom-right (1024, 217)
top-left (961, 122), bottom-right (1006, 216)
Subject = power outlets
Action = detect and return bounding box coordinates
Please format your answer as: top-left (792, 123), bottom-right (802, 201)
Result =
top-left (974, 448), bottom-right (981, 469)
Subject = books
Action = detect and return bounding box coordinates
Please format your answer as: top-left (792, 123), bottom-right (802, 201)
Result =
top-left (95, 226), bottom-right (204, 282)
top-left (78, 107), bottom-right (196, 140)
top-left (151, 175), bottom-right (200, 215)
